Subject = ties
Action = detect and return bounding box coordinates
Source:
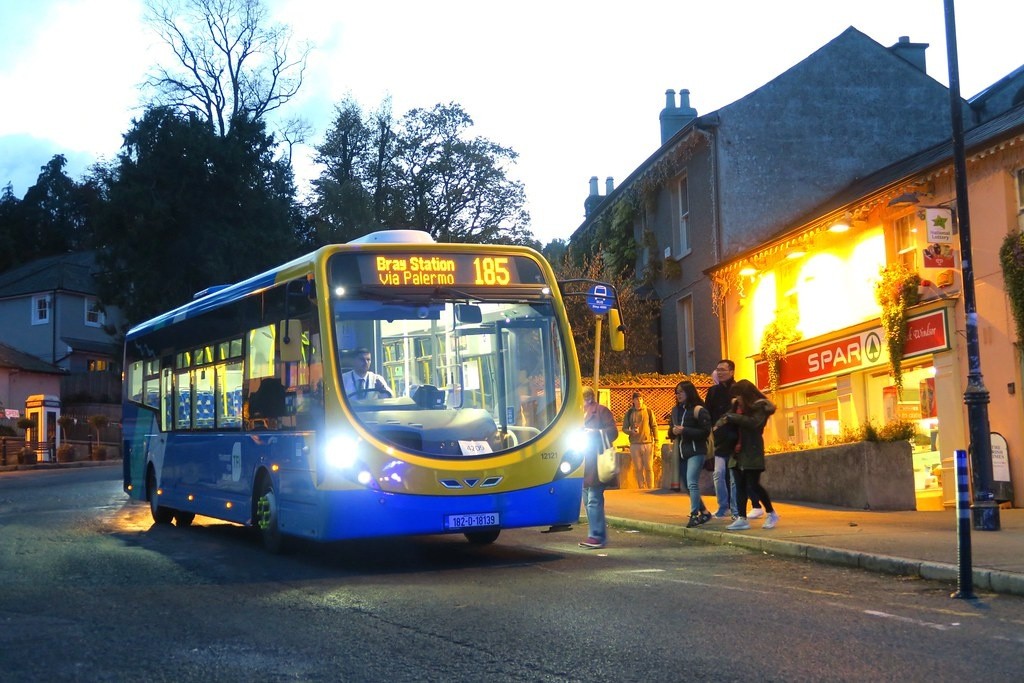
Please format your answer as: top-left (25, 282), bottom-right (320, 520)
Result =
top-left (358, 380), bottom-right (365, 399)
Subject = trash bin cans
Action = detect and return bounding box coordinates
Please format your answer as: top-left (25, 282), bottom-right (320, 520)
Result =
top-left (968, 432), bottom-right (1015, 508)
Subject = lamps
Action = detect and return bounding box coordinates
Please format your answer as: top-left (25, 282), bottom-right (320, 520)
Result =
top-left (785, 240), bottom-right (814, 259)
top-left (887, 183), bottom-right (935, 209)
top-left (738, 265), bottom-right (759, 275)
top-left (826, 218), bottom-right (868, 231)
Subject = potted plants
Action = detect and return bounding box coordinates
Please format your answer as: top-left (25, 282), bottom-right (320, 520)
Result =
top-left (55, 417), bottom-right (76, 462)
top-left (16, 418), bottom-right (39, 464)
top-left (88, 414), bottom-right (110, 460)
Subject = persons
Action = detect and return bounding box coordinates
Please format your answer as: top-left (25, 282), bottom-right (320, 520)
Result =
top-left (669, 381), bottom-right (711, 528)
top-left (704, 359), bottom-right (739, 521)
top-left (343, 347), bottom-right (393, 399)
top-left (712, 379), bottom-right (780, 529)
top-left (622, 392), bottom-right (659, 490)
top-left (251, 378), bottom-right (286, 418)
top-left (579, 387), bottom-right (618, 548)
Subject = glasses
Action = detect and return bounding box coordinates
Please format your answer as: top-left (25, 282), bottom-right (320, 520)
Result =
top-left (714, 367), bottom-right (730, 372)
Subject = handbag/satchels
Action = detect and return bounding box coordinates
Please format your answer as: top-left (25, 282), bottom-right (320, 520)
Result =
top-left (594, 427), bottom-right (620, 483)
top-left (712, 422), bottom-right (740, 457)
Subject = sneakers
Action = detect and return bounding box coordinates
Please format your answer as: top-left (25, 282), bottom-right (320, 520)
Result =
top-left (697, 512), bottom-right (712, 523)
top-left (725, 517), bottom-right (751, 529)
top-left (763, 511), bottom-right (778, 529)
top-left (687, 512), bottom-right (702, 527)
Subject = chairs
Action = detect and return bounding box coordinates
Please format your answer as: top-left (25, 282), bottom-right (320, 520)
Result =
top-left (134, 385), bottom-right (244, 428)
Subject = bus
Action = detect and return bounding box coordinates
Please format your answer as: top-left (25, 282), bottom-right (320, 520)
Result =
top-left (121, 229), bottom-right (628, 555)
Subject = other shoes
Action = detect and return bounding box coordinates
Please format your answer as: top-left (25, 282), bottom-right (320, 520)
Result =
top-left (731, 515), bottom-right (739, 521)
top-left (712, 511), bottom-right (728, 519)
top-left (747, 508), bottom-right (764, 521)
top-left (577, 538), bottom-right (607, 548)
